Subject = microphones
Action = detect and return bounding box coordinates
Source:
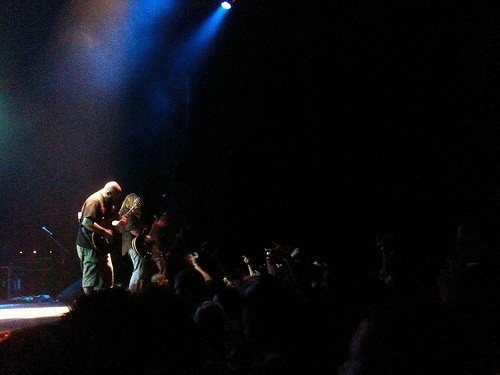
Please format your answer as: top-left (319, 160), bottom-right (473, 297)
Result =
top-left (42, 226), bottom-right (53, 235)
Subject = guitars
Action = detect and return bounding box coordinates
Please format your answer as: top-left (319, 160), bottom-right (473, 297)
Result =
top-left (131, 219), bottom-right (157, 260)
top-left (91, 198), bottom-right (141, 255)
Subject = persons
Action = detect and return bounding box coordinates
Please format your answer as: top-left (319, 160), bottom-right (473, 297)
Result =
top-left (76, 180), bottom-right (128, 294)
top-left (118, 193), bottom-right (153, 290)
top-left (148, 210), bottom-right (179, 284)
top-left (0, 226), bottom-right (500, 375)
